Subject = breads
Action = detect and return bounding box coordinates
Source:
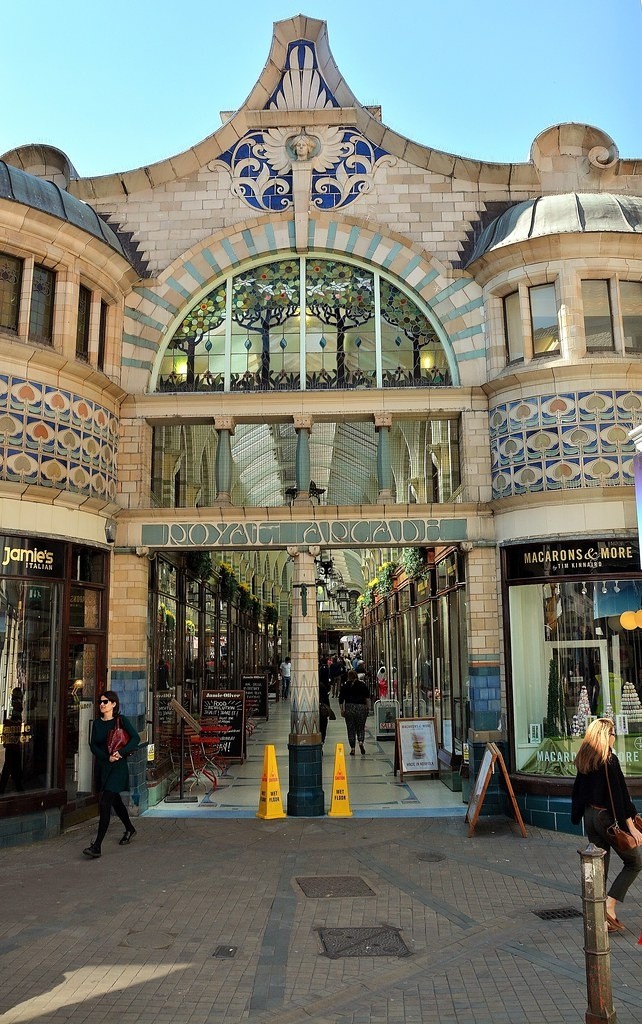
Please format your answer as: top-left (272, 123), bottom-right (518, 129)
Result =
top-left (412, 742), bottom-right (426, 759)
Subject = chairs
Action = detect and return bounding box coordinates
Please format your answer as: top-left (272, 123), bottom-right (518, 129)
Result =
top-left (169, 716), bottom-right (219, 795)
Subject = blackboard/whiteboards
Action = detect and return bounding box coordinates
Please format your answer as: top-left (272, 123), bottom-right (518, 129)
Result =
top-left (202, 690), bottom-right (247, 759)
top-left (259, 665), bottom-right (280, 703)
top-left (156, 690), bottom-right (191, 749)
top-left (239, 673), bottom-right (269, 719)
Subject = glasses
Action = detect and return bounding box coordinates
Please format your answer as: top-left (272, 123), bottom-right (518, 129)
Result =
top-left (611, 733), bottom-right (615, 736)
top-left (98, 699), bottom-right (112, 704)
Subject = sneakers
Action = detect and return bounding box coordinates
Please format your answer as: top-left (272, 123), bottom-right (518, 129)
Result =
top-left (119, 828), bottom-right (137, 845)
top-left (84, 843), bottom-right (102, 858)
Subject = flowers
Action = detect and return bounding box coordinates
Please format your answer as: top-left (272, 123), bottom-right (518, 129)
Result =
top-left (378, 561), bottom-right (396, 572)
top-left (159, 602), bottom-right (196, 630)
top-left (249, 593), bottom-right (273, 609)
top-left (218, 559), bottom-right (235, 574)
top-left (367, 577), bottom-right (381, 589)
top-left (233, 581), bottom-right (252, 593)
top-left (355, 592), bottom-right (365, 603)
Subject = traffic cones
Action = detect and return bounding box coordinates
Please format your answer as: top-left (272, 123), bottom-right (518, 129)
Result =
top-left (326, 743), bottom-right (354, 816)
top-left (255, 744), bottom-right (287, 820)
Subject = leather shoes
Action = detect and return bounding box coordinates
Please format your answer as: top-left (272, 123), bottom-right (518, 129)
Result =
top-left (606, 909), bottom-right (624, 932)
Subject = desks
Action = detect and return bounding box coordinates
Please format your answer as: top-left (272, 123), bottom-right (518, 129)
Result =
top-left (176, 735), bottom-right (220, 791)
top-left (188, 725), bottom-right (232, 775)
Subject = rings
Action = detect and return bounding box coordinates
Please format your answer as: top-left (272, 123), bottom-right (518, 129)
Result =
top-left (116, 755), bottom-right (118, 757)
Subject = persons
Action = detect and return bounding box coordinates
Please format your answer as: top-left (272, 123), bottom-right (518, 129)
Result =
top-left (572, 718), bottom-right (642, 933)
top-left (278, 653), bottom-right (291, 703)
top-left (82, 690), bottom-right (141, 858)
top-left (319, 639), bottom-right (371, 756)
top-left (1, 687), bottom-right (24, 793)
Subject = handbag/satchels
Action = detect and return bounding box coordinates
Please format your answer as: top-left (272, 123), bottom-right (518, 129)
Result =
top-left (107, 716), bottom-right (134, 756)
top-left (607, 816), bottom-right (642, 853)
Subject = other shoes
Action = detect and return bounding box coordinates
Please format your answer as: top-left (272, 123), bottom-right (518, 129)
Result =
top-left (350, 750), bottom-right (355, 755)
top-left (359, 743), bottom-right (365, 755)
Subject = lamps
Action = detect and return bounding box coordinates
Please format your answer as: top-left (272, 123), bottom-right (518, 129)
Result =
top-left (285, 488), bottom-right (298, 499)
top-left (620, 610), bottom-right (642, 631)
top-left (555, 580), bottom-right (620, 595)
top-left (309, 487), bottom-right (326, 497)
